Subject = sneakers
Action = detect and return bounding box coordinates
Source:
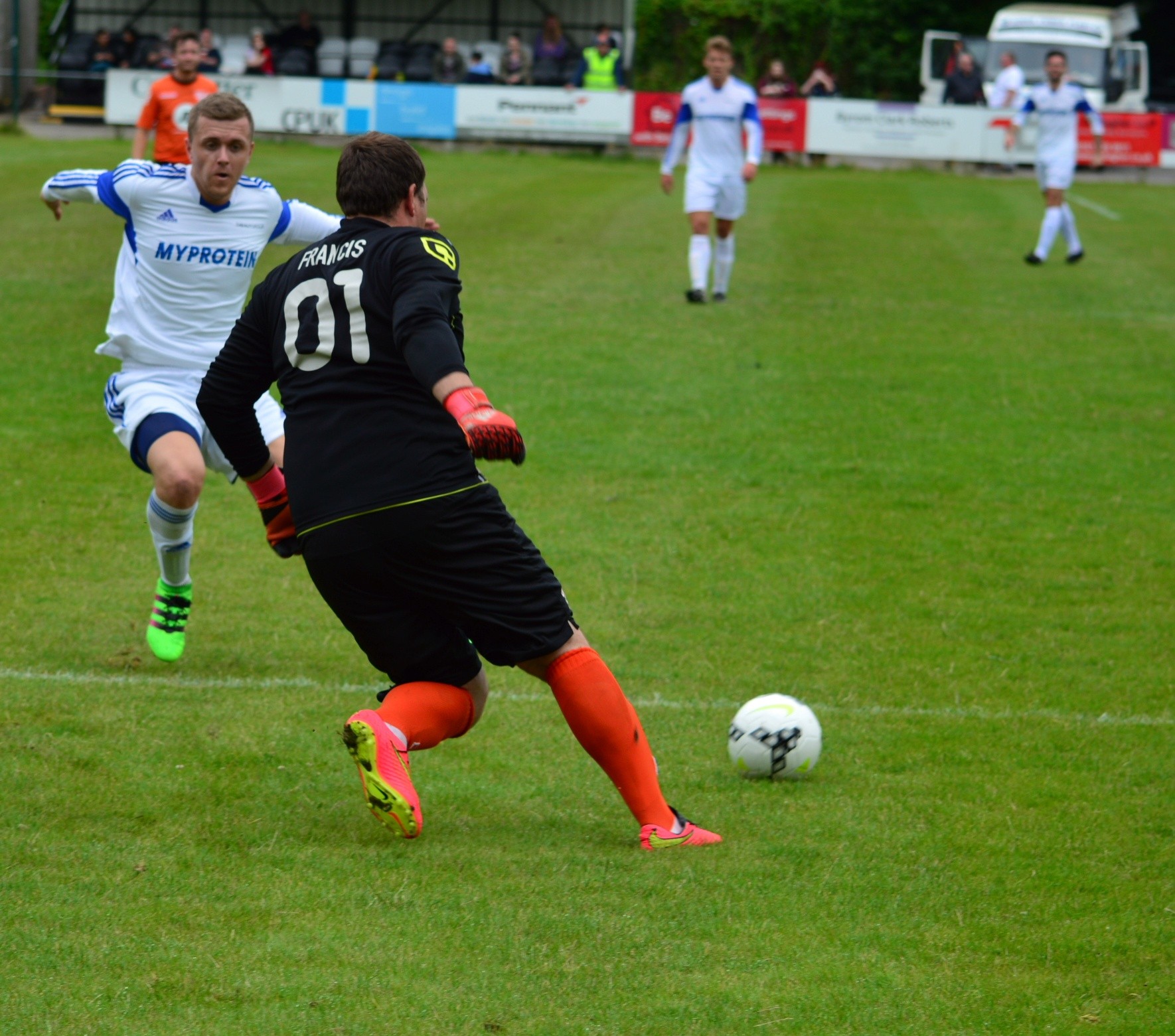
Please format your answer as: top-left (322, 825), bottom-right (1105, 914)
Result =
top-left (640, 806), bottom-right (722, 852)
top-left (146, 578), bottom-right (192, 661)
top-left (342, 708), bottom-right (423, 838)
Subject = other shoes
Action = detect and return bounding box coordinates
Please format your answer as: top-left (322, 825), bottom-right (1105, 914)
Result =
top-left (712, 292), bottom-right (725, 302)
top-left (1024, 250), bottom-right (1041, 264)
top-left (1066, 250), bottom-right (1084, 266)
top-left (685, 288), bottom-right (703, 303)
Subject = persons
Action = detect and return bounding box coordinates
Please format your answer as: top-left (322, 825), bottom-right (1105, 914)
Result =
top-left (1004, 49), bottom-right (1105, 265)
top-left (659, 35), bottom-right (763, 301)
top-left (195, 135), bottom-right (724, 849)
top-left (40, 94), bottom-right (439, 661)
top-left (85, 10), bottom-right (1023, 171)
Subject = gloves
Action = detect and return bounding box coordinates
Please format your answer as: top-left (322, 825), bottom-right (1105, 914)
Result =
top-left (247, 464), bottom-right (305, 560)
top-left (444, 386), bottom-right (525, 467)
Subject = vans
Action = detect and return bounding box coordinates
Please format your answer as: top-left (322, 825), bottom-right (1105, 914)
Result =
top-left (919, 3), bottom-right (1151, 172)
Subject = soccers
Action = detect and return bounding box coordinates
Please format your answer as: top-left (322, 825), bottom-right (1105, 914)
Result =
top-left (725, 690), bottom-right (823, 785)
